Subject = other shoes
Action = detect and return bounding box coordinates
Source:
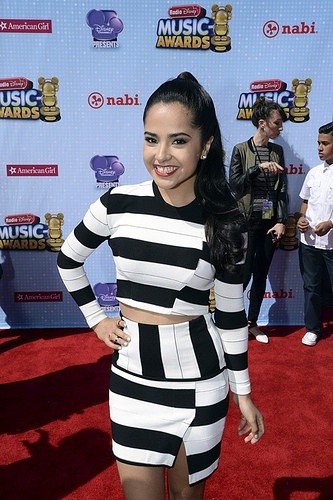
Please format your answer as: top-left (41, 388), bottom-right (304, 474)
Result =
top-left (249, 327), bottom-right (269, 344)
top-left (301, 332), bottom-right (318, 346)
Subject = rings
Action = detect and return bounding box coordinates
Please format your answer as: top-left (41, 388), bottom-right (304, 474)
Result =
top-left (117, 321), bottom-right (121, 325)
top-left (114, 336), bottom-right (119, 342)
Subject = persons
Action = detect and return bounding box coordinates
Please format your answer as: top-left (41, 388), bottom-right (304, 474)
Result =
top-left (297, 121), bottom-right (333, 349)
top-left (58, 72), bottom-right (265, 500)
top-left (230, 100), bottom-right (289, 344)
